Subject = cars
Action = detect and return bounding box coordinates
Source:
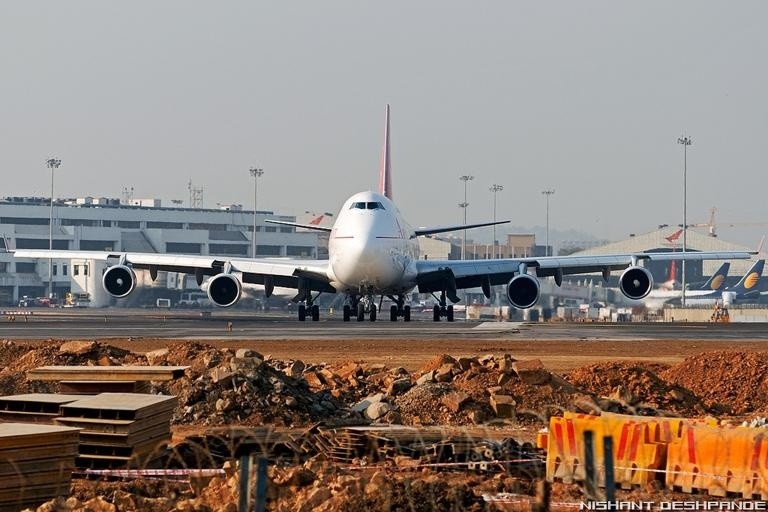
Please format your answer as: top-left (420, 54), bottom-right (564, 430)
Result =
top-left (179, 293), bottom-right (205, 308)
top-left (18, 291), bottom-right (90, 307)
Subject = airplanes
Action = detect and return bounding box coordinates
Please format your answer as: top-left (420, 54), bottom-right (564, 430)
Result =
top-left (13, 104), bottom-right (757, 321)
top-left (541, 260), bottom-right (766, 309)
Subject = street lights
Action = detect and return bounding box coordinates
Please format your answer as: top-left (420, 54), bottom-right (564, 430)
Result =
top-left (248, 167), bottom-right (263, 256)
top-left (677, 133), bottom-right (691, 309)
top-left (541, 189), bottom-right (552, 257)
top-left (43, 156), bottom-right (62, 300)
top-left (459, 175), bottom-right (473, 259)
top-left (490, 183), bottom-right (503, 261)
